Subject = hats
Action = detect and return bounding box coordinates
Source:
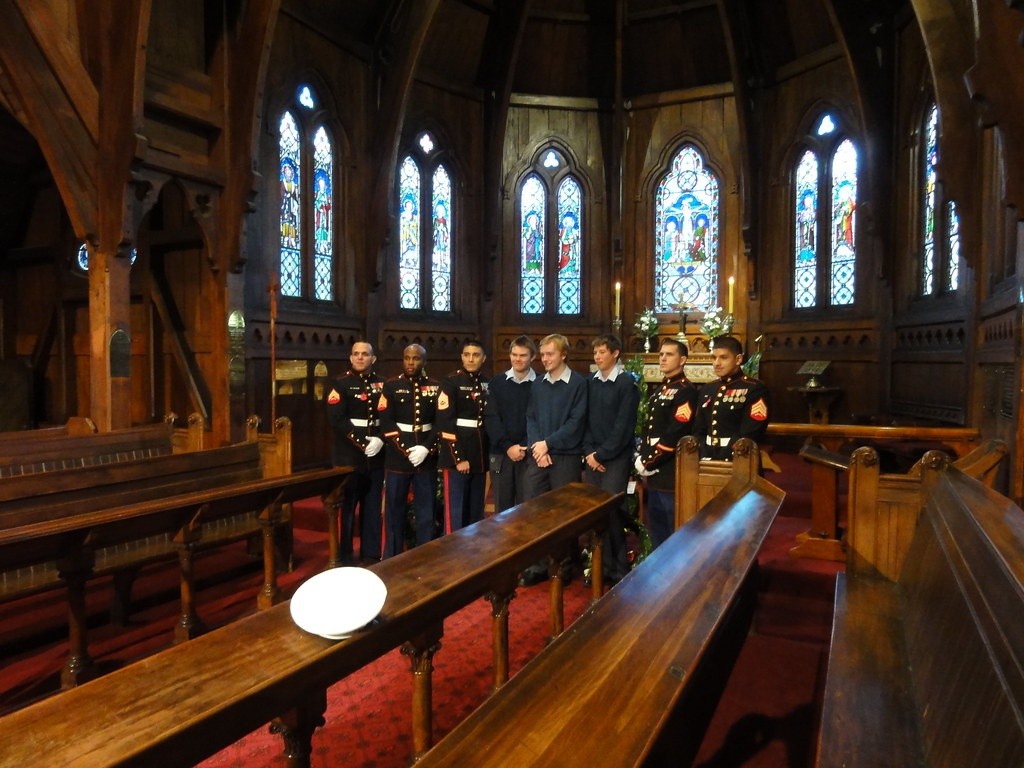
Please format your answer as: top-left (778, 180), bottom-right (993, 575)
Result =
top-left (289, 567), bottom-right (388, 640)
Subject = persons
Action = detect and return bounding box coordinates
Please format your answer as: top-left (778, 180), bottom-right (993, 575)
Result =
top-left (437, 340), bottom-right (490, 535)
top-left (581, 334), bottom-right (641, 590)
top-left (483, 336), bottom-right (540, 515)
top-left (377, 344), bottom-right (444, 562)
top-left (519, 333), bottom-right (588, 587)
top-left (327, 340), bottom-right (388, 566)
top-left (634, 339), bottom-right (697, 551)
top-left (693, 337), bottom-right (771, 477)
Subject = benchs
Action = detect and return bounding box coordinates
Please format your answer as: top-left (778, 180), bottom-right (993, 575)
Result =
top-left (3, 412), bottom-right (1021, 766)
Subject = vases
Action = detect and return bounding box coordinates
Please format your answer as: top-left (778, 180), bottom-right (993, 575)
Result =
top-left (709, 337), bottom-right (714, 354)
top-left (644, 337), bottom-right (650, 353)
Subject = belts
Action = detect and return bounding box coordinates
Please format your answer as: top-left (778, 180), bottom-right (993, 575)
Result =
top-left (350, 418), bottom-right (380, 427)
top-left (706, 435), bottom-right (731, 447)
top-left (397, 423), bottom-right (432, 432)
top-left (640, 438), bottom-right (660, 446)
top-left (456, 418), bottom-right (478, 428)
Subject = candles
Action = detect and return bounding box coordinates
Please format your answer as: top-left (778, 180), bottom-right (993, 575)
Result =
top-left (616, 283), bottom-right (620, 317)
top-left (728, 276), bottom-right (733, 313)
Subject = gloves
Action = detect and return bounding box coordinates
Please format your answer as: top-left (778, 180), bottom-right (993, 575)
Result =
top-left (406, 445), bottom-right (430, 467)
top-left (364, 436), bottom-right (384, 457)
top-left (635, 455), bottom-right (659, 476)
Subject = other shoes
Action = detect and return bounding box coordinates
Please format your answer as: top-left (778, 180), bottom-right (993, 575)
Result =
top-left (520, 569), bottom-right (549, 587)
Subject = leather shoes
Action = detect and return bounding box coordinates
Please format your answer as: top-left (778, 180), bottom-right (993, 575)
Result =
top-left (584, 578), bottom-right (591, 587)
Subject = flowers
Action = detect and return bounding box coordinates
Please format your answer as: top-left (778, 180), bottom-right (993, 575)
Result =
top-left (700, 305), bottom-right (731, 338)
top-left (634, 309), bottom-right (658, 337)
